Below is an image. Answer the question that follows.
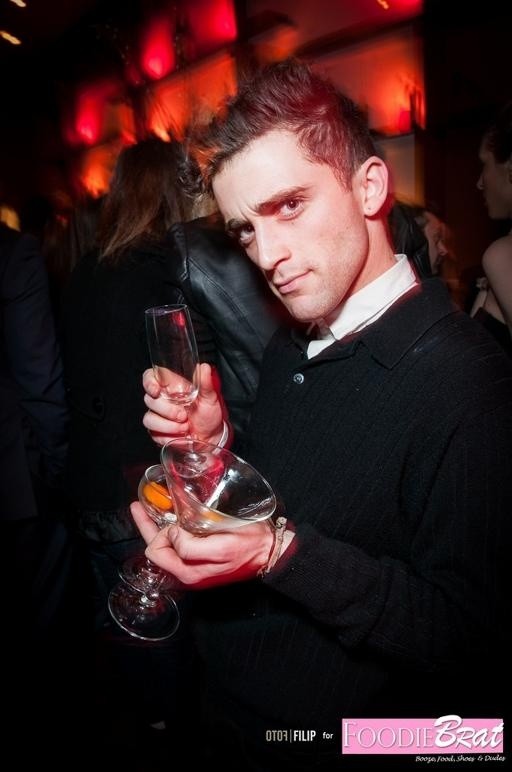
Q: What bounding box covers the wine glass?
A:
[107,437,278,642]
[144,303,223,479]
